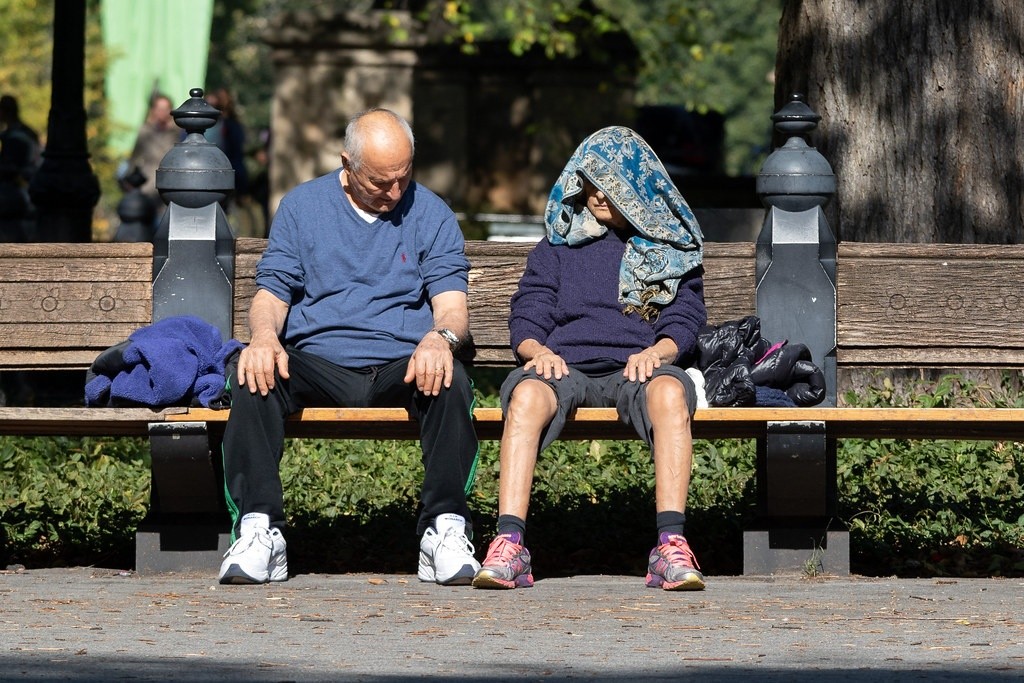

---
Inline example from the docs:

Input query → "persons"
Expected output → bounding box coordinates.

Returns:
[215,108,480,588]
[0,82,275,242]
[466,126,709,592]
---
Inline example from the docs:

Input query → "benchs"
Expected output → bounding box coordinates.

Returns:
[0,87,1023,578]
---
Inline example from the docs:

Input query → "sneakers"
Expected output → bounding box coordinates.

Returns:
[217,511,289,583]
[471,533,535,589]
[416,512,482,585]
[644,533,707,591]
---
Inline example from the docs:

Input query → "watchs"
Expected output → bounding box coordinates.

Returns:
[431,325,460,353]
